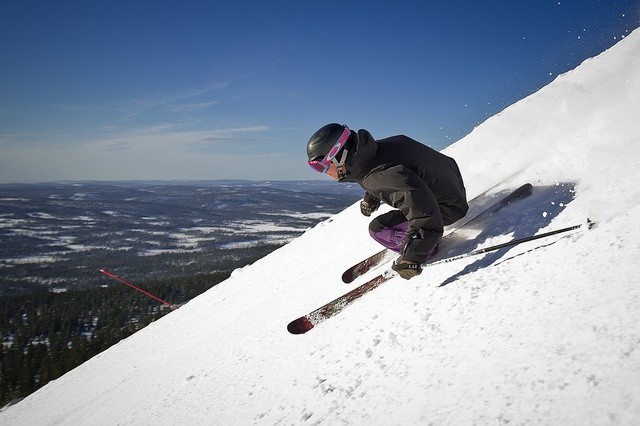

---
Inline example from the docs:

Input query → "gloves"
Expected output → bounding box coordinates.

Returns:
[360,202,376,216]
[395,258,422,280]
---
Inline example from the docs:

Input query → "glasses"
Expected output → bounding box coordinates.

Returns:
[308,125,351,174]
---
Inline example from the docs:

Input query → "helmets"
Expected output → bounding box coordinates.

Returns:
[307,123,357,176]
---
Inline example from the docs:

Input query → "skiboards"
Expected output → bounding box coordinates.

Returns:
[287,165,533,335]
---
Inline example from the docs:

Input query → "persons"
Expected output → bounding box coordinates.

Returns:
[307,122,468,280]
